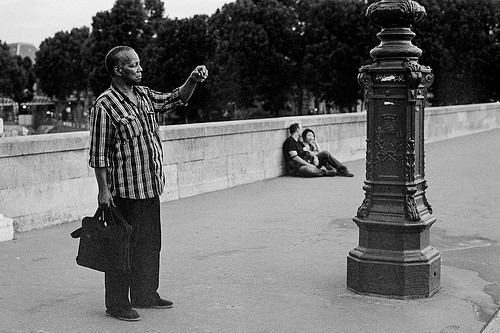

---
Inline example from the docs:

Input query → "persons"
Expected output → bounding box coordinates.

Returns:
[283,123,355,177]
[89,46,208,321]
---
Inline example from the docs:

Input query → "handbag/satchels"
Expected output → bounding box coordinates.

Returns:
[311,156,323,169]
[70,204,133,275]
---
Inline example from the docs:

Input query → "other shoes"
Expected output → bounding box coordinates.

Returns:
[105,307,141,320]
[336,166,354,177]
[326,164,333,170]
[327,170,336,176]
[131,299,174,308]
